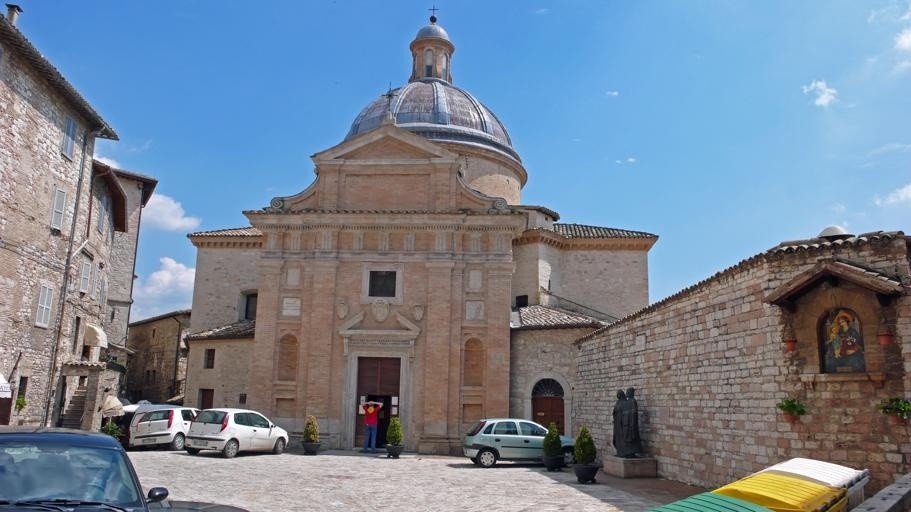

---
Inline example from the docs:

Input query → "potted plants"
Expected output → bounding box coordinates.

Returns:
[538,422,568,471]
[567,425,604,483]
[301,415,322,455]
[385,416,405,458]
[778,400,806,423]
[878,398,911,425]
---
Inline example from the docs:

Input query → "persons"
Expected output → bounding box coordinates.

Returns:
[822,308,865,373]
[359,400,384,454]
[612,389,629,458]
[618,387,642,458]
[826,321,848,362]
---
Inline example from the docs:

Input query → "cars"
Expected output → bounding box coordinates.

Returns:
[0,425,169,512]
[129,404,289,458]
[463,418,575,468]
